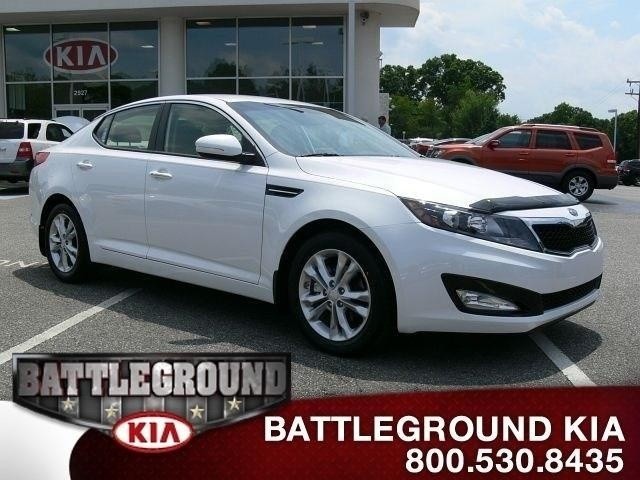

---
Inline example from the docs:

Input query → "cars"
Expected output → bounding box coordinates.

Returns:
[403,132,489,153]
[24,93,604,356]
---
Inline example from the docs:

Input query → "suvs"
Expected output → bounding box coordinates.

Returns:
[1,116,91,185]
[424,122,618,203]
[618,160,639,187]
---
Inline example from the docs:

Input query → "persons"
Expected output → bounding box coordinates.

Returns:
[376,115,393,136]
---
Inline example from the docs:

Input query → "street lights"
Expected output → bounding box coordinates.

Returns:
[609,109,617,154]
